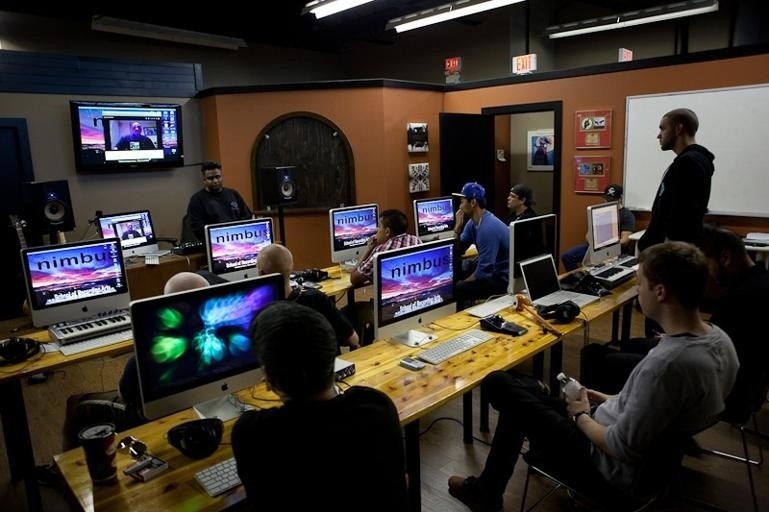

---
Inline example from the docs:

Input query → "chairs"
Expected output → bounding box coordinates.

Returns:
[676,365,763,512]
[521,420,682,511]
[62,387,146,449]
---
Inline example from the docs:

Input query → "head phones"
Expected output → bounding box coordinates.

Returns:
[559,270,613,297]
[0,336,39,367]
[535,300,580,325]
[289,267,328,285]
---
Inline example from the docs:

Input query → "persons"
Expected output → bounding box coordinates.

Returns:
[506,187,544,220]
[602,227,768,415]
[114,121,157,150]
[562,185,637,271]
[230,303,410,512]
[444,183,514,307]
[255,245,360,354]
[638,109,715,339]
[121,221,140,238]
[115,271,213,416]
[444,241,742,512]
[340,210,425,324]
[183,162,254,234]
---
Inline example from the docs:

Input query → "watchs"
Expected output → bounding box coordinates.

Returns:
[571,411,590,425]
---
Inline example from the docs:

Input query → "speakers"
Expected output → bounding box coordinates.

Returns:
[256,166,299,209]
[19,179,77,240]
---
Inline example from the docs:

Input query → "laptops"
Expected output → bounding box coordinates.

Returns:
[519,253,601,314]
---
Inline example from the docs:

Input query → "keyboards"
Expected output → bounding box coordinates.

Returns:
[137,249,171,256]
[145,255,160,265]
[582,255,643,290]
[464,294,519,318]
[416,328,495,365]
[58,328,134,356]
[193,457,243,497]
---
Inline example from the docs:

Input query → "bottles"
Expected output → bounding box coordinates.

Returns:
[557,373,599,419]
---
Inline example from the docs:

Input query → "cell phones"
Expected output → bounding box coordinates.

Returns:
[400,357,426,371]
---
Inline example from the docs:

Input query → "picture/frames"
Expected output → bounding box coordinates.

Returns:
[574,155,611,194]
[527,129,554,171]
[575,109,612,149]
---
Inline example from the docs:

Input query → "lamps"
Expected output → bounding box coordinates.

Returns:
[386,0,527,33]
[543,0,719,40]
[91,16,247,50]
[301,0,373,20]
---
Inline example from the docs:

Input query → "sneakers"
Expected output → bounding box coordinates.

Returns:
[535,378,552,401]
[448,473,505,511]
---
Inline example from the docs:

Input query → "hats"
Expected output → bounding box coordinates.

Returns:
[511,183,536,205]
[603,185,623,199]
[452,183,486,200]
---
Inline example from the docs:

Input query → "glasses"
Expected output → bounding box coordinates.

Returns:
[117,436,146,458]
[509,193,518,199]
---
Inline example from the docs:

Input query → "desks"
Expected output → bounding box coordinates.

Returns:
[0,244,481,512]
[123,243,203,301]
[628,229,769,269]
[52,256,641,512]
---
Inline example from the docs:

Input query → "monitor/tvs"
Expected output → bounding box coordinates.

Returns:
[97,209,157,258]
[413,195,457,241]
[507,214,559,295]
[69,99,184,176]
[581,201,621,267]
[129,271,286,424]
[373,236,457,348]
[20,237,131,345]
[204,217,275,283]
[329,203,379,273]
